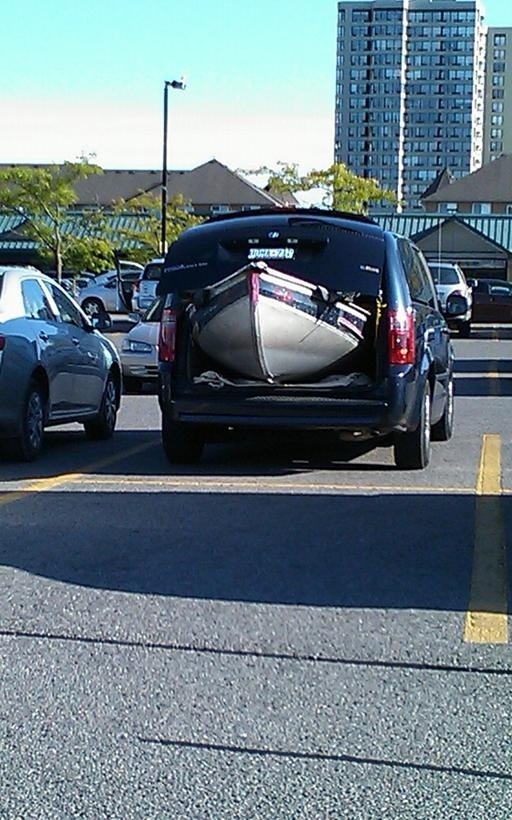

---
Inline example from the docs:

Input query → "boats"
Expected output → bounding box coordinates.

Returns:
[185,260,372,386]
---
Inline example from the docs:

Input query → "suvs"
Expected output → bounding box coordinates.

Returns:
[426,262,477,335]
[158,207,469,472]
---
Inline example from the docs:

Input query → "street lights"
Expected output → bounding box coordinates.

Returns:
[161,79,187,255]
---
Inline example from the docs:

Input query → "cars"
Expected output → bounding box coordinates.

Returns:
[44,259,166,395]
[0,264,125,460]
[468,277,512,323]
